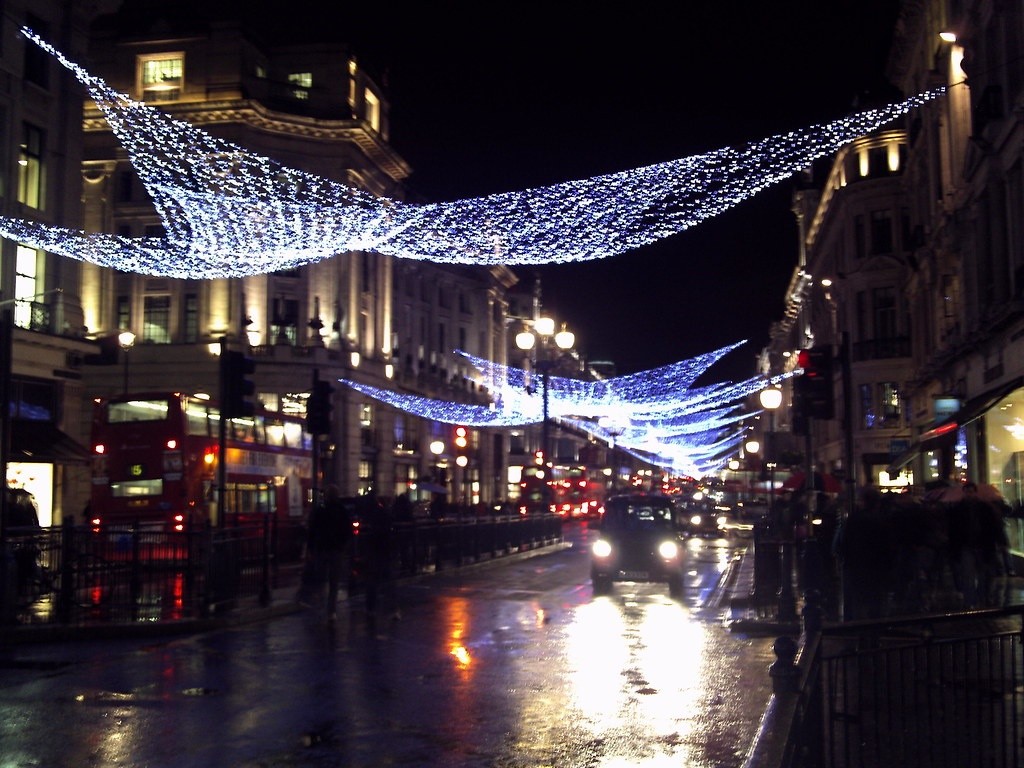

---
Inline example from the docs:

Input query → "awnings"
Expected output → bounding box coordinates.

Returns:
[420,483,448,493]
[6,417,93,466]
[885,376,1024,480]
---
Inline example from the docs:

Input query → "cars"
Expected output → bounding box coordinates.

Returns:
[670,496,719,537]
[585,492,688,584]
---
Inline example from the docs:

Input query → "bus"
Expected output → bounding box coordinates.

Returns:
[83,389,323,563]
[521,461,606,519]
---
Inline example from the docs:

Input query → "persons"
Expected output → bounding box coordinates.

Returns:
[308,484,351,617]
[775,481,1018,622]
[372,493,448,577]
[6,488,41,603]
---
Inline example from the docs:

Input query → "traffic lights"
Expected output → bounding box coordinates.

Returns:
[305,390,332,435]
[223,350,257,418]
[797,344,834,419]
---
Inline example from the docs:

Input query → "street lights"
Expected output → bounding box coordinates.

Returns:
[514,316,576,514]
[759,376,782,551]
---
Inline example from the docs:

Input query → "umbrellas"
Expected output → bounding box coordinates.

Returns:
[783,472,845,492]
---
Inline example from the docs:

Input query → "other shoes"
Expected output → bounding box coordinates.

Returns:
[386,612,401,622]
[298,599,313,609]
[328,612,338,622]
